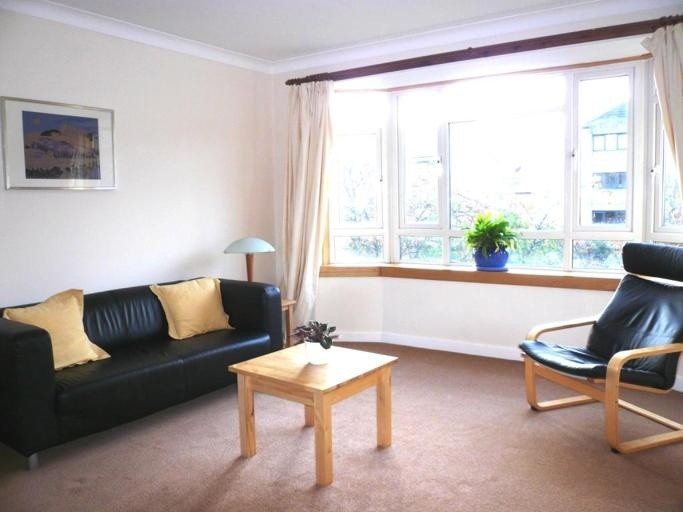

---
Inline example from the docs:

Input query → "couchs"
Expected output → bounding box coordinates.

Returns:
[0,278,282,472]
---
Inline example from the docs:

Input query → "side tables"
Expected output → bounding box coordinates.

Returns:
[281,300,297,347]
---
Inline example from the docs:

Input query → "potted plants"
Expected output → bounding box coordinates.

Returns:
[463,211,520,271]
[293,320,340,366]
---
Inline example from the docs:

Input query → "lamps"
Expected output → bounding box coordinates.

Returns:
[224,236,276,282]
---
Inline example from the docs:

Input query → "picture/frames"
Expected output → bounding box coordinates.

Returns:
[0,95,116,192]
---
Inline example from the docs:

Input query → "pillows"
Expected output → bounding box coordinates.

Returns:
[148,278,236,340]
[3,289,111,372]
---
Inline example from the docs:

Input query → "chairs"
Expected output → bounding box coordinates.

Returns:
[518,242,683,454]
